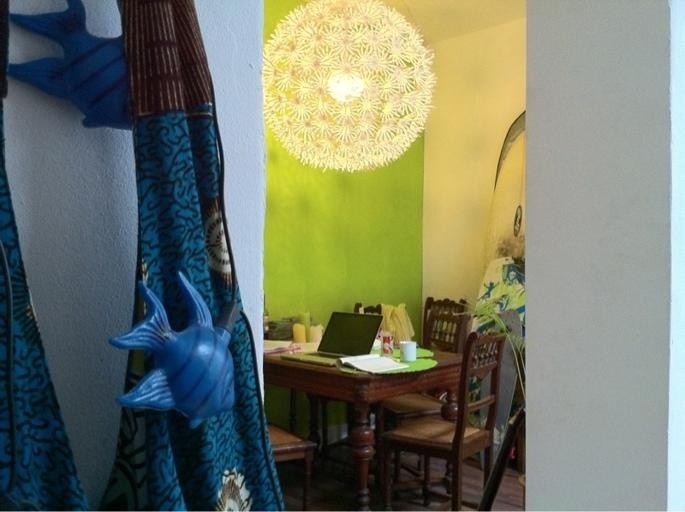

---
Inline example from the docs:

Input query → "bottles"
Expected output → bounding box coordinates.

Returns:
[262,294,269,338]
[380,331,393,358]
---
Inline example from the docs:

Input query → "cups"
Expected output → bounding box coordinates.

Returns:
[399,340,416,362]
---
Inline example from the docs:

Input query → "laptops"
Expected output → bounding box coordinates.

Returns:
[280,311,384,367]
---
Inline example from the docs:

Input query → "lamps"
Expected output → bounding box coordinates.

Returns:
[261,2,436,175]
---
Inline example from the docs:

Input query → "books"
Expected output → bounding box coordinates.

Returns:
[336,353,410,376]
